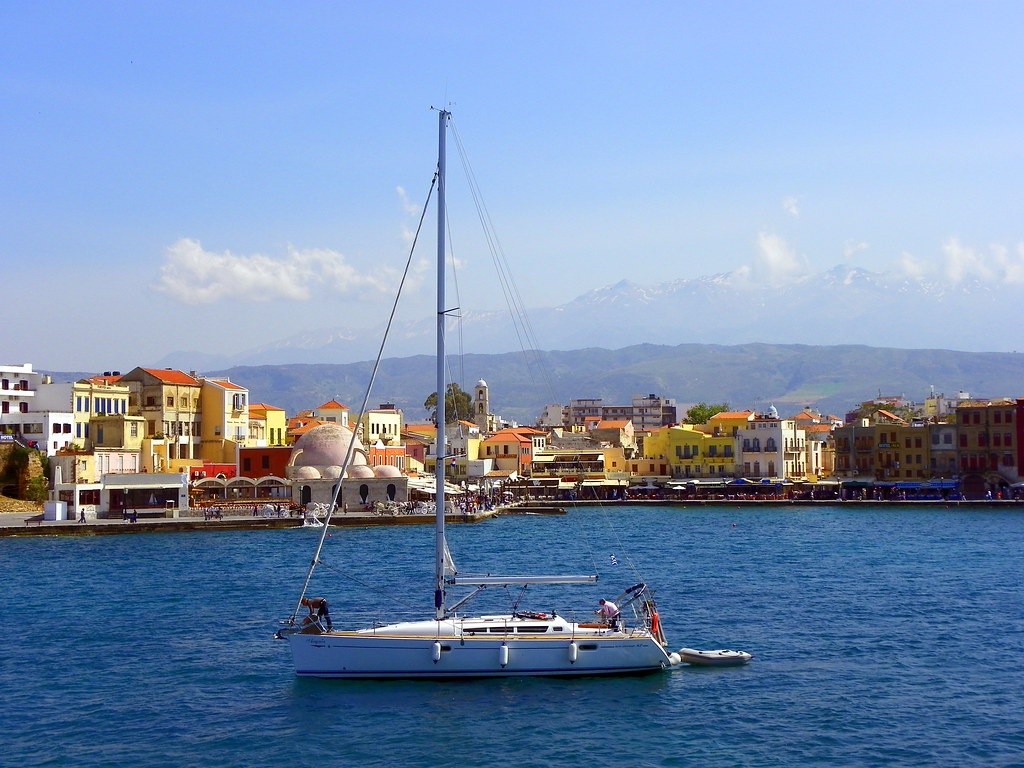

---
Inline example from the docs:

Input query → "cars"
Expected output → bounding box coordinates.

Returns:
[0,431,40,454]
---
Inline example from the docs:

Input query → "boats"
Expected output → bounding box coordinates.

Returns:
[678,647,756,667]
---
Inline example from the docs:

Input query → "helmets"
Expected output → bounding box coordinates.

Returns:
[300,597,308,603]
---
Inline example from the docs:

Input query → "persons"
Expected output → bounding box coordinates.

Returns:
[204,504,223,520]
[78,508,86,523]
[810,490,814,500]
[986,488,1024,500]
[133,510,137,522]
[859,486,905,501]
[736,492,739,499]
[344,502,348,515]
[277,504,280,517]
[603,488,628,501]
[458,492,509,515]
[253,503,258,516]
[301,597,334,633]
[595,599,621,628]
[366,499,418,515]
[962,492,966,501]
[290,504,306,517]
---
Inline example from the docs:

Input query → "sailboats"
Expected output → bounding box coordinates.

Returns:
[272,100,682,682]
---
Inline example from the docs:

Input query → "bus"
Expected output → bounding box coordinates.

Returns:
[529,459,608,483]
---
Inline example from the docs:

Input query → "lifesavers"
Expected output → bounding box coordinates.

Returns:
[652,613,658,632]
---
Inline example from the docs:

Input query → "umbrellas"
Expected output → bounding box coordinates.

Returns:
[628,485,659,494]
[673,485,686,496]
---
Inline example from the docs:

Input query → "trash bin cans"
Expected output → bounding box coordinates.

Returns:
[839,489,846,501]
[166,500,175,518]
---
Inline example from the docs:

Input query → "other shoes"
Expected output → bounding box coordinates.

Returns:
[327,626,333,633]
